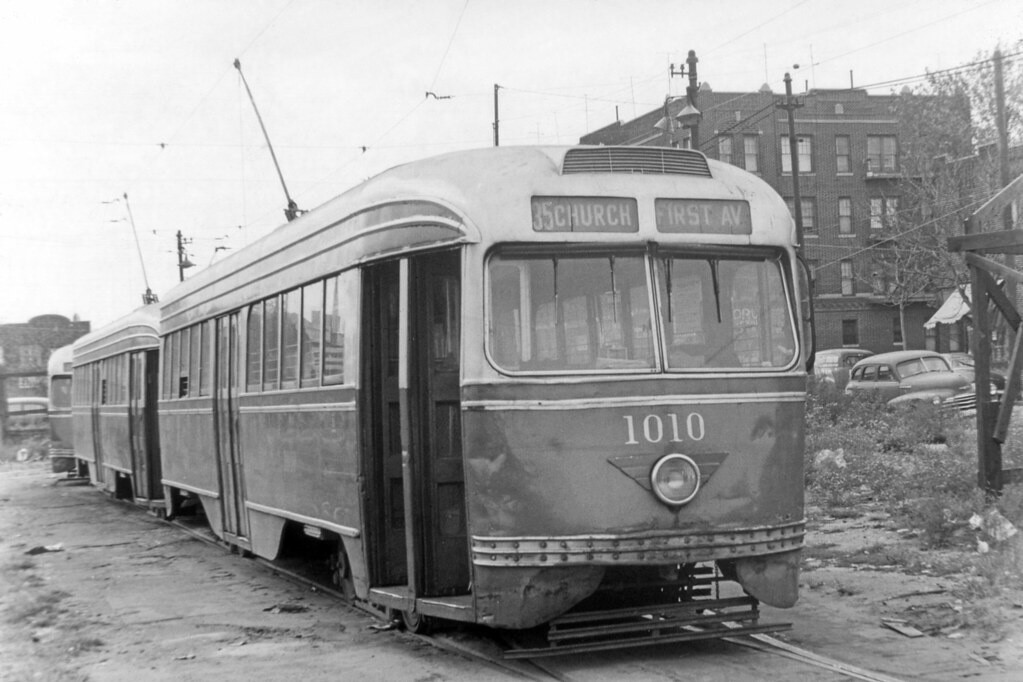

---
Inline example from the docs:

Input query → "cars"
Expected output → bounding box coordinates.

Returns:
[808,348,1006,426]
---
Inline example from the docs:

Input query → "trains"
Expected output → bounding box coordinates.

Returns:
[49,141,811,662]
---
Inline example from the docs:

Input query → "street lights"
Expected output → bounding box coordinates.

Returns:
[655,49,704,150]
